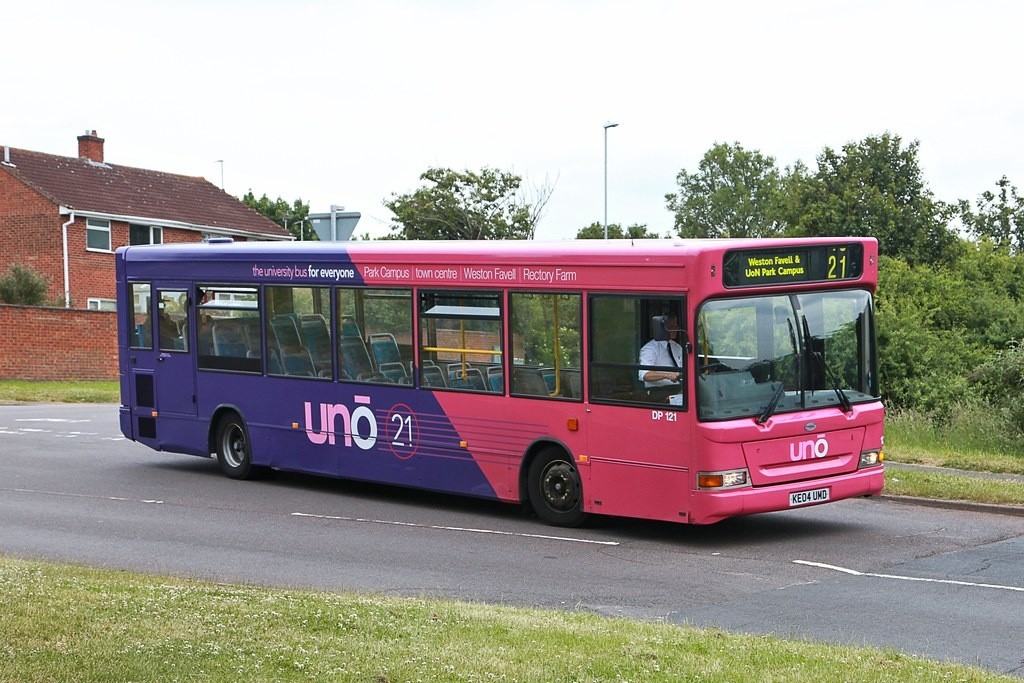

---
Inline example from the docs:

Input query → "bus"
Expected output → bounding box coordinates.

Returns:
[115,235,892,530]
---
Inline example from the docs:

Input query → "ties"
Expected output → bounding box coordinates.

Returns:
[667,342,678,367]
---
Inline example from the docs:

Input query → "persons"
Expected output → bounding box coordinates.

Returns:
[142,297,222,354]
[637,307,682,404]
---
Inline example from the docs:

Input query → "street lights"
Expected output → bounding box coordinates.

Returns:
[603,120,621,242]
[218,159,225,191]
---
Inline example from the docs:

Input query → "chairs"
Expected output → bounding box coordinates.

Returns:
[137,314,565,396]
